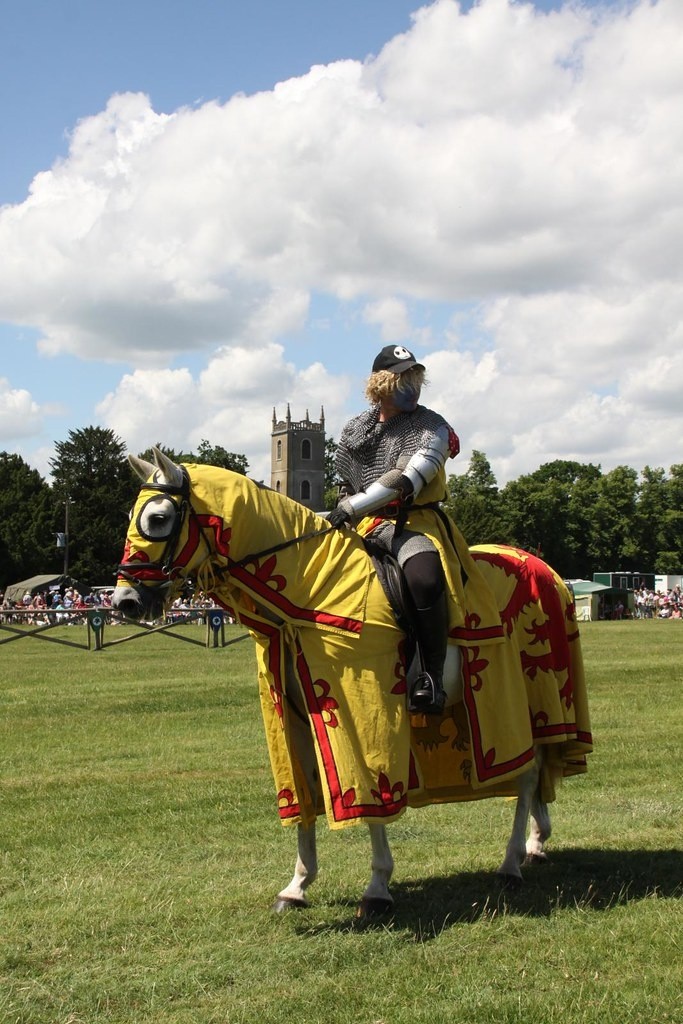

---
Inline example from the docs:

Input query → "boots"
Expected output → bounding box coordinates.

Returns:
[410,591,448,716]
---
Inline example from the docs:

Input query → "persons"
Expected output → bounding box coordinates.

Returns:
[604,584,683,620]
[325,344,459,715]
[0,587,236,625]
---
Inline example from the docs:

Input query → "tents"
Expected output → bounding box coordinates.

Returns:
[2,574,92,606]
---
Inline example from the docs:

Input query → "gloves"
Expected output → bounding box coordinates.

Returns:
[324,506,351,529]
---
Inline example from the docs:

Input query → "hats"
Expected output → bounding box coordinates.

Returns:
[371,344,426,374]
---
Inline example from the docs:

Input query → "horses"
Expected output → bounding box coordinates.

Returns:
[109,444,597,921]
